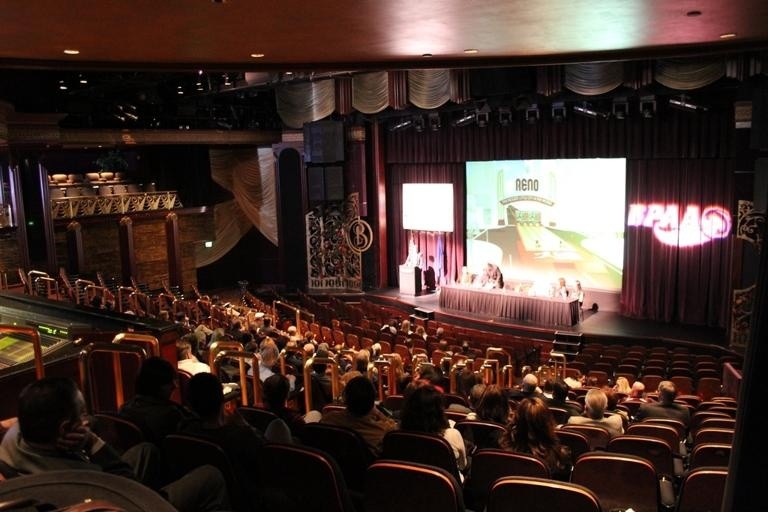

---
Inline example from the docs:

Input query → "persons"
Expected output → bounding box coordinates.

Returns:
[403,245,422,271]
[0,272,696,512]
[481,263,505,290]
